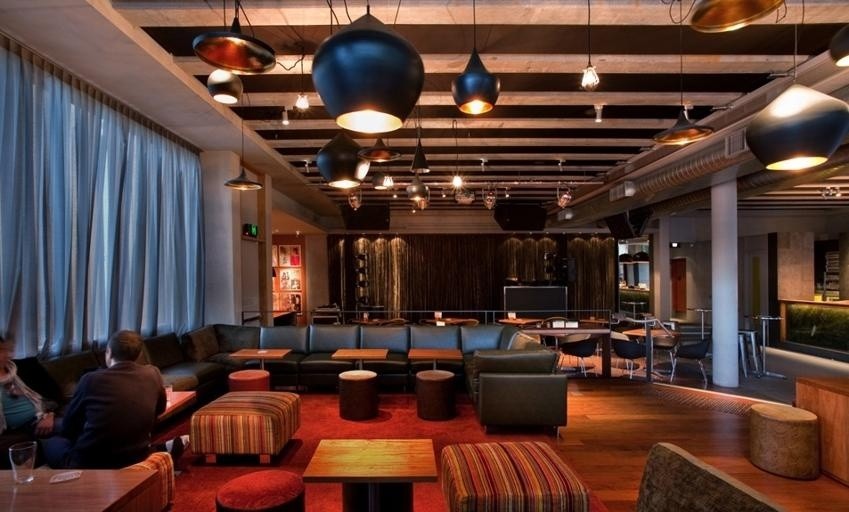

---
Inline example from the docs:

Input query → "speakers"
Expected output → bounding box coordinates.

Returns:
[493,204,548,232]
[341,204,390,231]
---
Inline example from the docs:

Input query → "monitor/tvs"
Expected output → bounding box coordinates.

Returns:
[505,285,568,321]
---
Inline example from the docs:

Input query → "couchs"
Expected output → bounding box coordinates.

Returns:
[247,323,505,391]
[464,326,569,440]
[46,326,260,422]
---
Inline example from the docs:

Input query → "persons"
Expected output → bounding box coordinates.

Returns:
[0,333,45,470]
[33,330,168,468]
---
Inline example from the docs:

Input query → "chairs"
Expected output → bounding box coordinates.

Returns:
[558,337,598,377]
[668,336,709,390]
[541,317,569,329]
[612,338,647,379]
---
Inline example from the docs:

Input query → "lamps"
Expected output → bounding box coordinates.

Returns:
[192,1,276,74]
[312,0,424,136]
[376,163,397,192]
[453,188,476,205]
[503,190,509,197]
[554,182,573,209]
[651,0,715,146]
[451,2,501,116]
[746,25,848,171]
[280,107,292,125]
[481,183,497,210]
[578,0,602,90]
[411,100,432,174]
[619,245,633,265]
[303,160,311,171]
[204,68,242,104]
[557,159,565,171]
[292,42,310,112]
[225,107,263,192]
[407,178,426,211]
[357,135,401,163]
[314,129,371,190]
[830,23,849,68]
[687,1,783,33]
[633,246,650,263]
[449,120,463,188]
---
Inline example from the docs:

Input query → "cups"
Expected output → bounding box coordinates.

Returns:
[9,441,39,483]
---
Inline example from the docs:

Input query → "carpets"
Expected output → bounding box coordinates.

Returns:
[158,391,610,511]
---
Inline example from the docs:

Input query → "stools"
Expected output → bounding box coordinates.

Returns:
[101,451,180,509]
[749,402,821,478]
[443,440,589,512]
[216,471,304,512]
[227,370,270,394]
[339,370,377,419]
[190,391,300,464]
[417,370,455,419]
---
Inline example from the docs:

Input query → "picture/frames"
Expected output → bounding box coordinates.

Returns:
[271,267,280,292]
[272,244,278,267]
[279,292,303,313]
[277,267,302,292]
[273,292,280,312]
[278,243,303,268]
